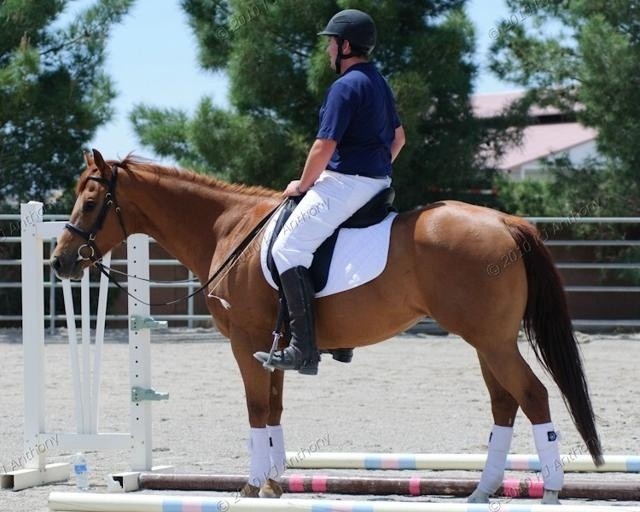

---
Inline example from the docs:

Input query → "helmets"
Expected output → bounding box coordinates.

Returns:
[316,9,379,50]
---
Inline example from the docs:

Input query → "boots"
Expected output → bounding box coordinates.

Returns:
[319,349,354,363]
[252,265,319,376]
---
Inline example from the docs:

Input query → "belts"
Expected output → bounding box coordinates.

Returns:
[351,173,391,180]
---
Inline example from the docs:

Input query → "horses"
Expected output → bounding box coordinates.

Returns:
[49,149,607,505]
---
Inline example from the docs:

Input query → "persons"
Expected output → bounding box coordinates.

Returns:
[252,9,406,375]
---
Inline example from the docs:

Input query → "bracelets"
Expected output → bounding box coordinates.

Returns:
[296,186,304,195]
